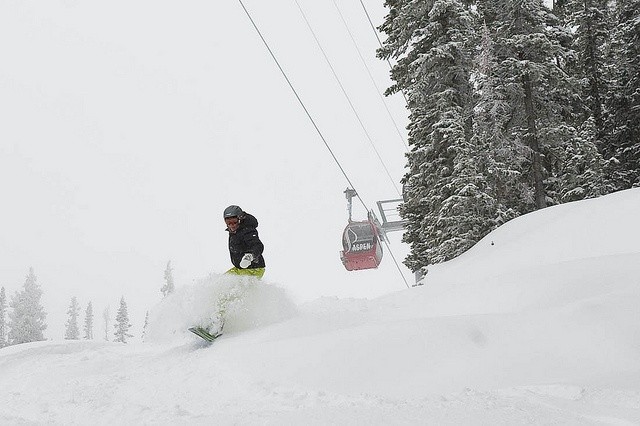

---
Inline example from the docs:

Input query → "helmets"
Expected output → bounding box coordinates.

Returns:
[224,205,246,220]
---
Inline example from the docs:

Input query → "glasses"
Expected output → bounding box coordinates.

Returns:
[225,217,238,225]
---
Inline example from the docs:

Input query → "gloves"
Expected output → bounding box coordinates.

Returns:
[240,253,253,269]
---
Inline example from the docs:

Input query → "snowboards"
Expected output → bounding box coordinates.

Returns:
[188,325,222,342]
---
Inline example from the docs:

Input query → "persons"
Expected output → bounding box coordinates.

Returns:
[206,206,266,336]
